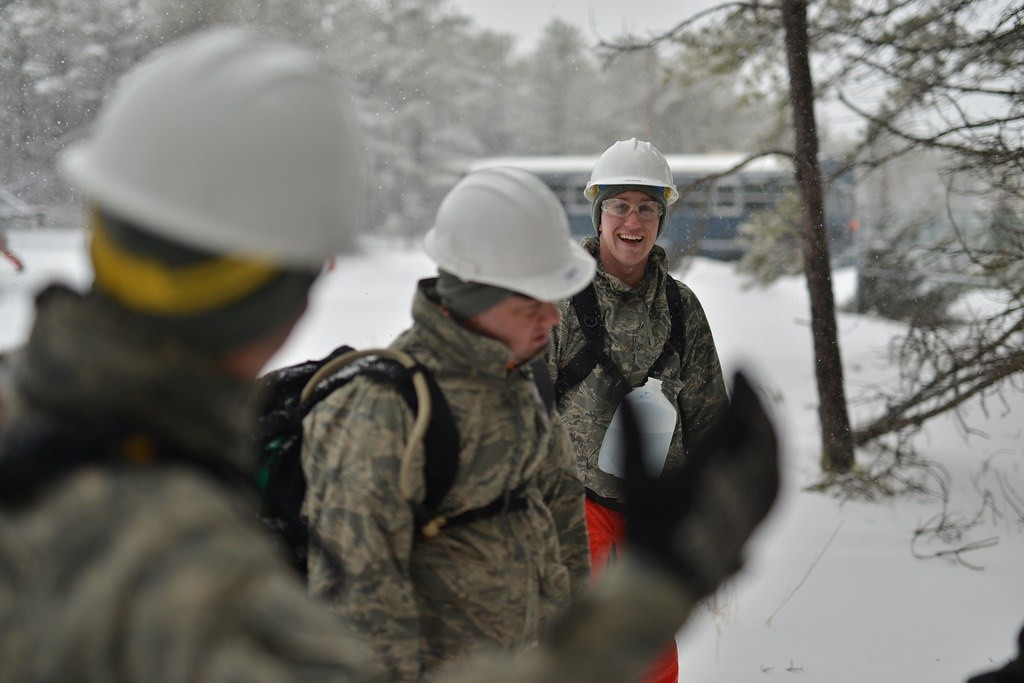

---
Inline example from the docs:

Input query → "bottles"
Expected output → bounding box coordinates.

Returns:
[597,377,678,483]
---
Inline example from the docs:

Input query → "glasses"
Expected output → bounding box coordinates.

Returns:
[601,198,664,220]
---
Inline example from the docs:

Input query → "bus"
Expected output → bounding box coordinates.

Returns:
[462,145,859,262]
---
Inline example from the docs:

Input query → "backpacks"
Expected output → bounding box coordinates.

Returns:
[243,346,556,582]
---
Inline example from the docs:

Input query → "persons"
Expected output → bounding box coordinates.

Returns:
[298,165,593,683]
[1,26,781,683]
[546,139,729,682]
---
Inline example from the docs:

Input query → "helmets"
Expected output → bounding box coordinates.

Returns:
[423,168,597,304]
[584,138,679,207]
[56,26,372,273]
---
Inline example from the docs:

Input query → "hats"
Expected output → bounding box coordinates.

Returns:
[591,185,670,239]
[435,268,511,324]
